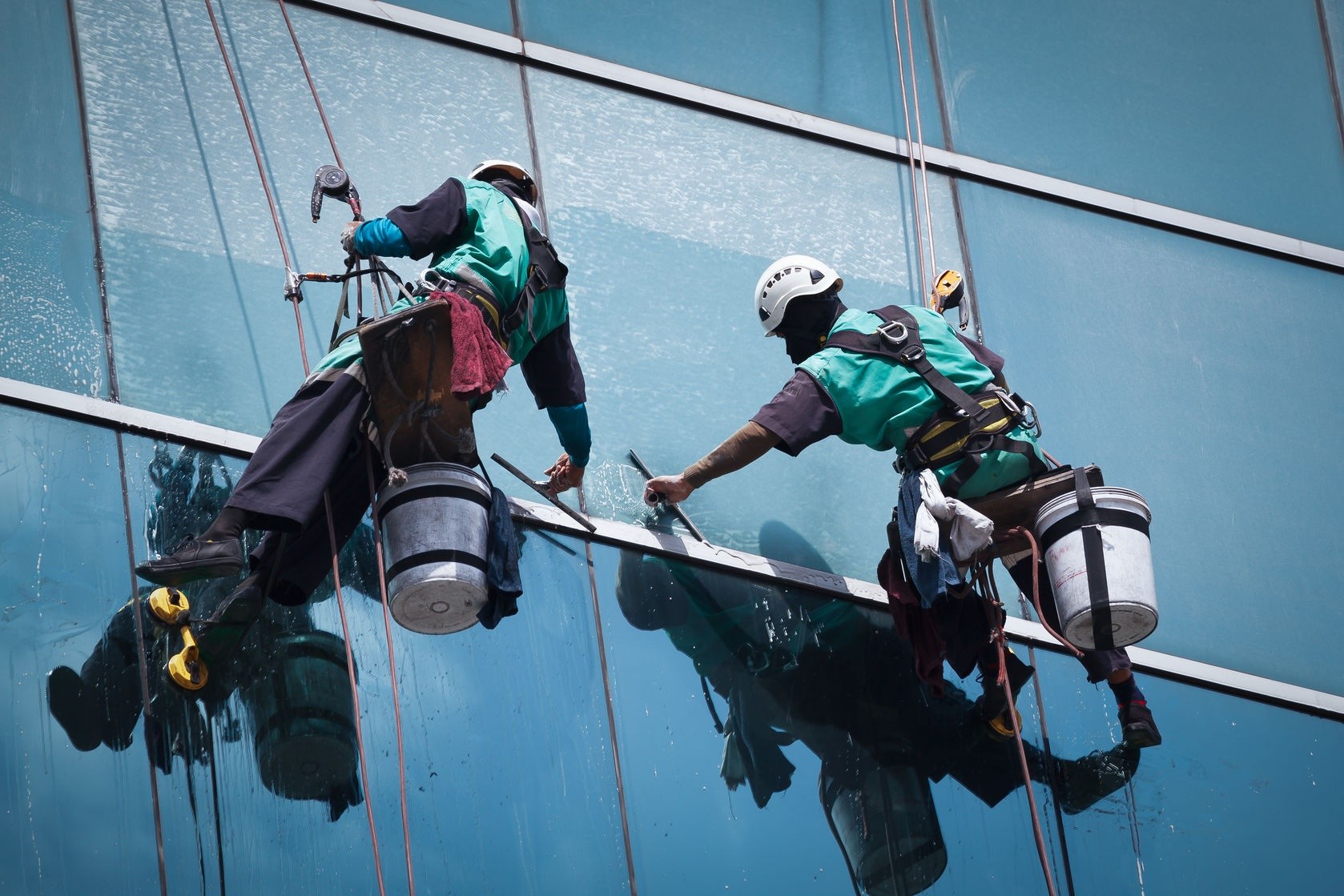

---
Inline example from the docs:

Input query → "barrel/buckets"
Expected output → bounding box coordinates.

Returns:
[240,632,358,799]
[373,462,492,636]
[817,741,948,896]
[1035,487,1157,649]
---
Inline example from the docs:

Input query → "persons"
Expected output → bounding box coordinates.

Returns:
[617,510,1141,816]
[135,159,592,672]
[644,253,1162,748]
[45,487,389,775]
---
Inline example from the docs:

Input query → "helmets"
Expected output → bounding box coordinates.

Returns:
[467,159,538,209]
[755,254,845,338]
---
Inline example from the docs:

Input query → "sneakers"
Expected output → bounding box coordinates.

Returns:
[46,664,133,750]
[1063,744,1141,816]
[978,655,1033,715]
[134,539,242,586]
[1116,704,1163,747]
[207,588,259,650]
